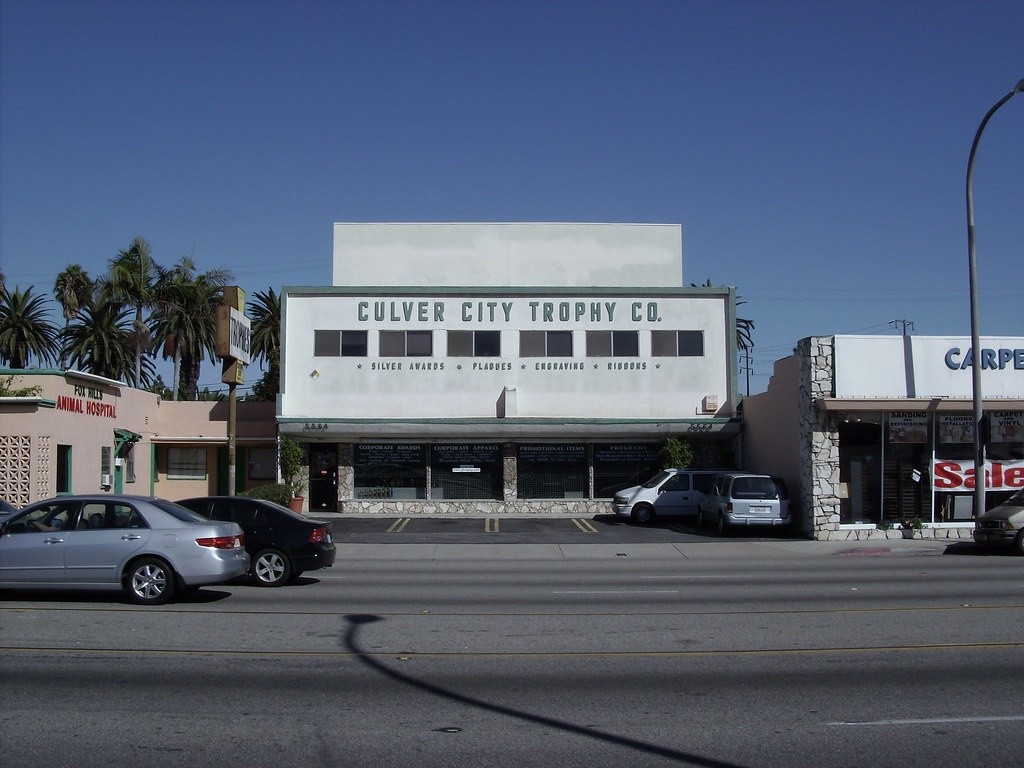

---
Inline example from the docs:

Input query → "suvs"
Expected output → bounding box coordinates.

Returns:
[698,473,793,536]
[595,464,663,499]
[610,468,753,526]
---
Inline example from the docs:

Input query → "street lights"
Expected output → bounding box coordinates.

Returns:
[969,77,1024,530]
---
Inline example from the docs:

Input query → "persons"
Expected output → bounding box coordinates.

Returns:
[26,505,76,531]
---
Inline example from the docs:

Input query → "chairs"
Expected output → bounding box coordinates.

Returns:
[89,513,104,529]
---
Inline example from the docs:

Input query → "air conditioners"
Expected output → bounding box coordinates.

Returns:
[102,474,113,486]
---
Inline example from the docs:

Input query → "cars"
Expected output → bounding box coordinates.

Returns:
[0,494,250,606]
[116,496,336,588]
[973,486,1024,555]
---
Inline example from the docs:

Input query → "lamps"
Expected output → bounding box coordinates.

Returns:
[140,431,160,437]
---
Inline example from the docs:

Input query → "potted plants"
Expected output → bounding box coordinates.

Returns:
[274,434,308,513]
